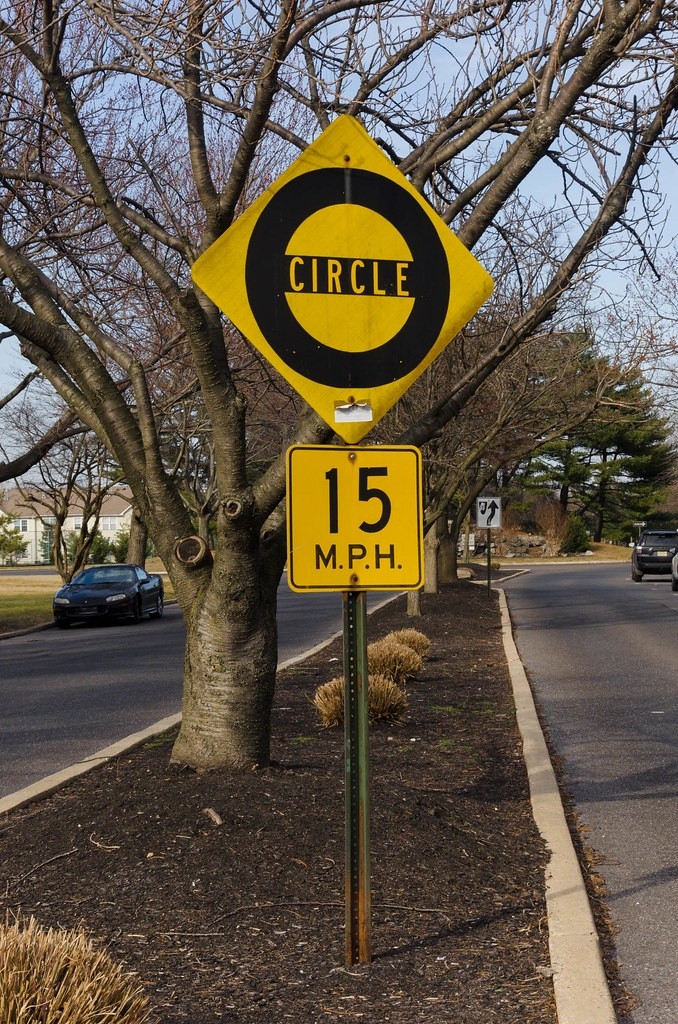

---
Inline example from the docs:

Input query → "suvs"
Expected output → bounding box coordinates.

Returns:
[631,528,678,582]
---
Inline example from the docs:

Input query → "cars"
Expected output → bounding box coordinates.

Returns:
[672,553,678,591]
[53,564,164,629]
